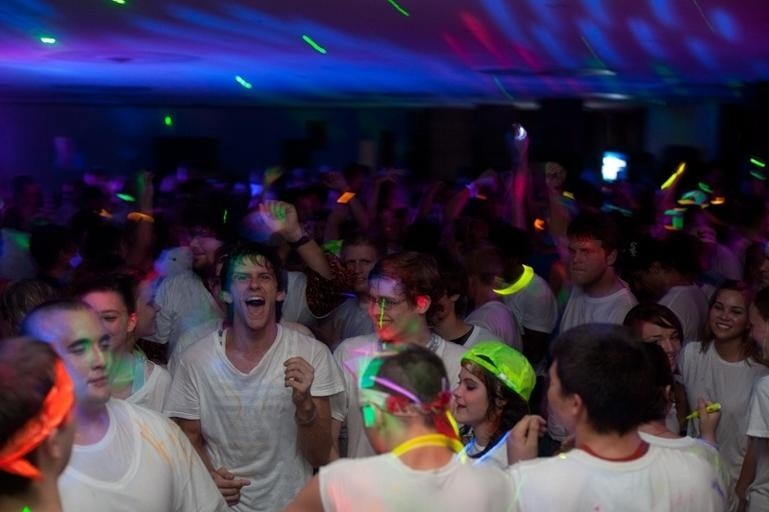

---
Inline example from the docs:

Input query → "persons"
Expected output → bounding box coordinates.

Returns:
[1,164,769,512]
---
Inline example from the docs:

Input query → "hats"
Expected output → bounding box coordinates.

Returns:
[461,341,537,414]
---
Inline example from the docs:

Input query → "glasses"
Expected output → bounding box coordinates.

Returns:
[365,295,405,311]
